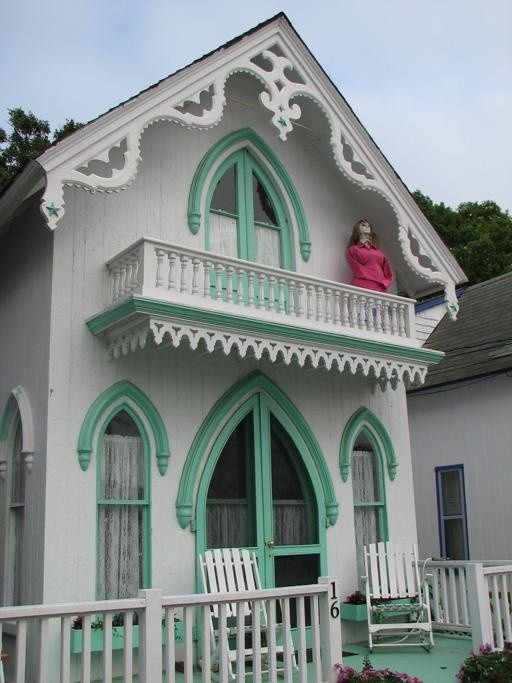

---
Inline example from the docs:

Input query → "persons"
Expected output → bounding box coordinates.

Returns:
[347,219,392,291]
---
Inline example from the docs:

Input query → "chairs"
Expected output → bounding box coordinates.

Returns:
[362,538,435,654]
[199,547,300,683]
